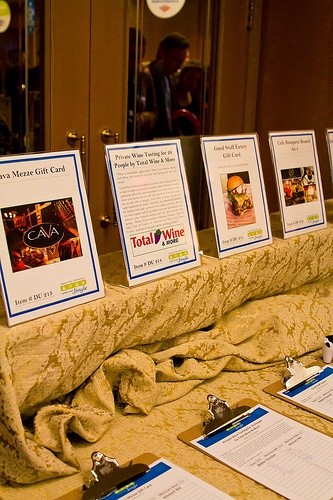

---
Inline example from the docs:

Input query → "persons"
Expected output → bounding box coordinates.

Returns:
[3,50,27,143]
[127,26,203,142]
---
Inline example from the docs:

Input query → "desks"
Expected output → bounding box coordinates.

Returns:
[0,198,333,489]
